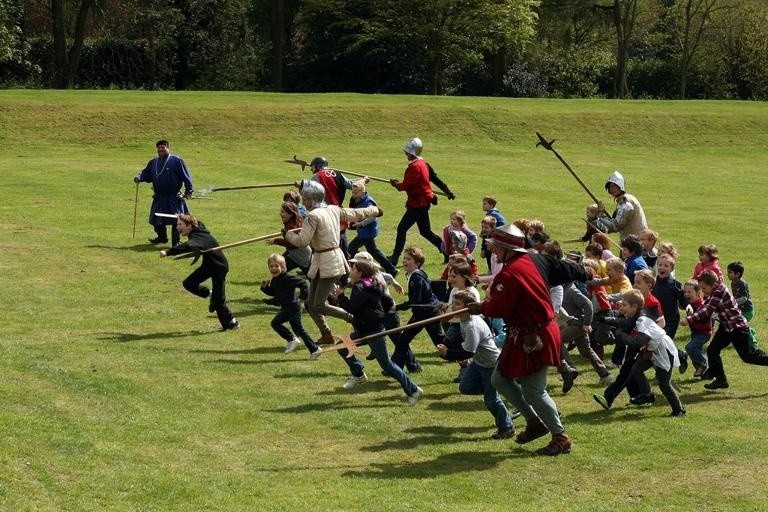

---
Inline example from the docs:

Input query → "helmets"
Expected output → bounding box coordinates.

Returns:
[310,158,327,169]
[605,170,626,195]
[298,180,329,209]
[484,223,531,254]
[405,137,424,158]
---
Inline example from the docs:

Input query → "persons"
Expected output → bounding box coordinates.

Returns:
[256,156,768,457]
[157,213,241,333]
[134,138,194,248]
[384,135,456,264]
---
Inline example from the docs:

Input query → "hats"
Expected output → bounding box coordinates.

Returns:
[353,179,366,194]
[157,140,169,147]
[348,252,373,264]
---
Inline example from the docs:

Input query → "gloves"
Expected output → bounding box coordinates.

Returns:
[448,192,456,200]
[390,178,400,187]
[378,208,384,218]
[585,265,596,281]
[467,302,482,315]
[281,228,288,237]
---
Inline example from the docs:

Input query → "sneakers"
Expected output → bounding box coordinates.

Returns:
[384,255,399,283]
[566,331,615,360]
[453,368,467,383]
[593,393,609,409]
[262,297,280,308]
[489,409,521,439]
[627,391,656,408]
[408,387,423,407]
[284,337,300,354]
[223,321,240,332]
[209,302,215,313]
[694,363,730,389]
[315,332,333,345]
[679,351,688,373]
[344,313,356,330]
[670,410,687,417]
[309,348,321,360]
[148,237,168,244]
[366,352,423,375]
[343,374,367,388]
[596,360,622,388]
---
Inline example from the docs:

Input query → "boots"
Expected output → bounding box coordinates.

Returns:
[515,415,549,444]
[536,430,571,456]
[557,361,578,393]
